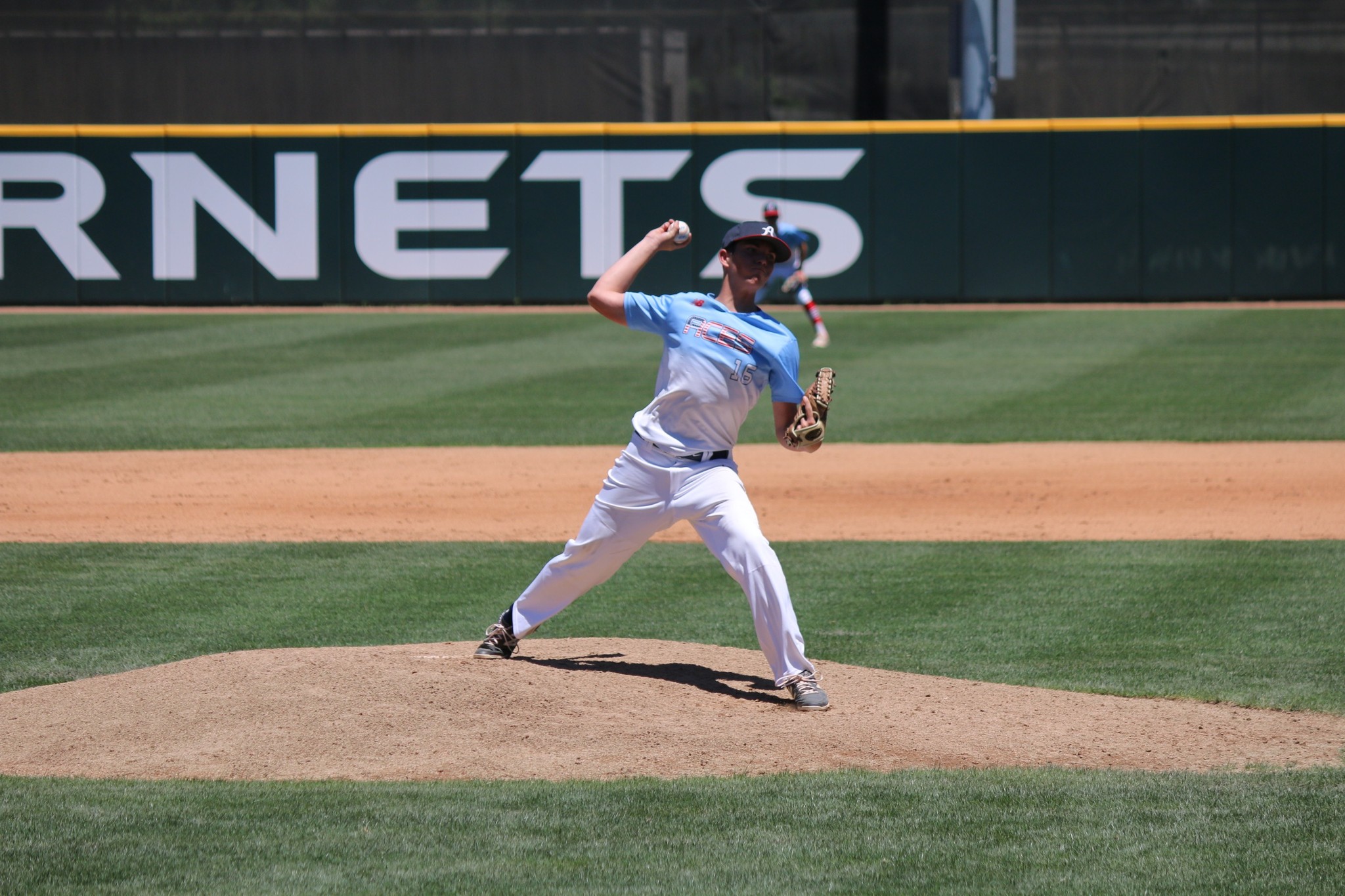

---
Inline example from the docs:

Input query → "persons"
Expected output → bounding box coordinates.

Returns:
[753,200,832,350]
[472,218,836,712]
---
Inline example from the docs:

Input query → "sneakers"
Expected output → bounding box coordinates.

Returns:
[774,668,831,712]
[473,609,520,661]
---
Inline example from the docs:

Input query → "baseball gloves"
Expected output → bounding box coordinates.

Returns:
[780,275,801,292]
[784,367,835,448]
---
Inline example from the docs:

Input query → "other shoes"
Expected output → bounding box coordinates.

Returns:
[810,331,833,350]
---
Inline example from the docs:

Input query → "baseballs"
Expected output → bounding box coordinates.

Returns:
[668,221,689,245]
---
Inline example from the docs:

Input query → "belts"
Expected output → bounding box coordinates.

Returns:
[634,429,729,463]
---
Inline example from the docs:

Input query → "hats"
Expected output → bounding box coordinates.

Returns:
[721,221,792,263]
[763,202,780,217]
